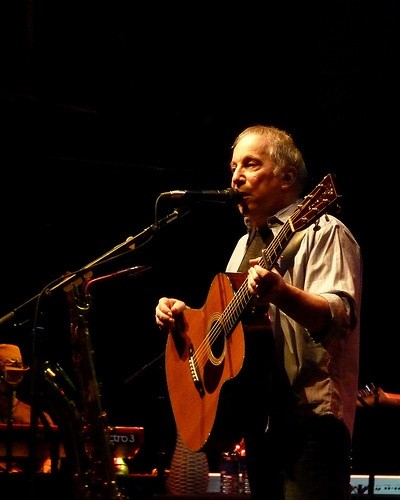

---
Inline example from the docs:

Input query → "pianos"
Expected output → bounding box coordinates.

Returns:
[0,424,144,461]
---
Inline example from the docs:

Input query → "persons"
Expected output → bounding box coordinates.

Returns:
[156,125,360,500]
[0,343,66,474]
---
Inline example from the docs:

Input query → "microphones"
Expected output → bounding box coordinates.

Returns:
[162,187,243,206]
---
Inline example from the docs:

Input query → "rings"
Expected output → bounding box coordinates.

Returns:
[256,285,259,287]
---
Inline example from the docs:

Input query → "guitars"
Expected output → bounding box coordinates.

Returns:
[163,172,337,454]
